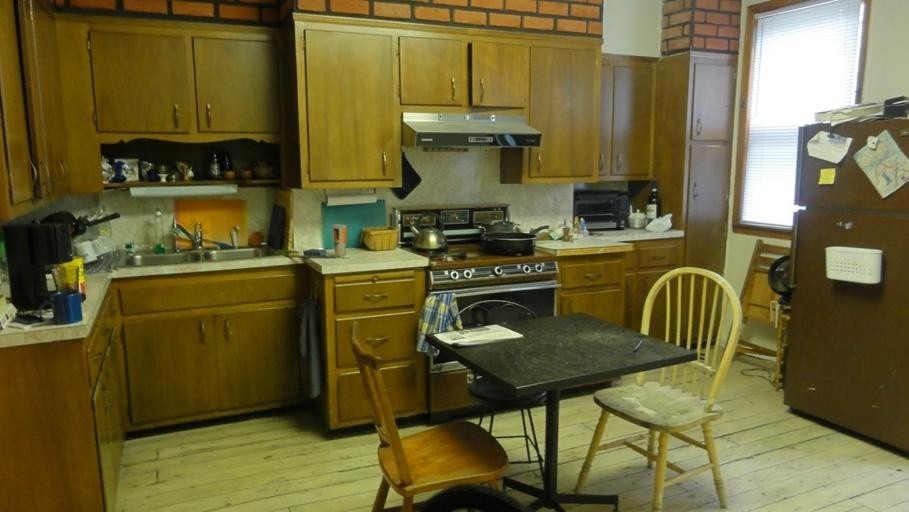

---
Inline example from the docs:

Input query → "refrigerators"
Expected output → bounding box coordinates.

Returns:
[785,116,909,456]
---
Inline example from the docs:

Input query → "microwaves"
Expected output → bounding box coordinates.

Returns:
[574,192,627,231]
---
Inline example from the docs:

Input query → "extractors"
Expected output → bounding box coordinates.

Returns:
[401,111,542,149]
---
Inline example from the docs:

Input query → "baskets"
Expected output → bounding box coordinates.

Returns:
[360,226,401,252]
[824,245,883,285]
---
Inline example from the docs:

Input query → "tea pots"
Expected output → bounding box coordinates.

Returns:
[411,210,448,253]
[72,239,104,266]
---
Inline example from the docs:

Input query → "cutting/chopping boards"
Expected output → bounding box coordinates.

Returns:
[267,188,295,252]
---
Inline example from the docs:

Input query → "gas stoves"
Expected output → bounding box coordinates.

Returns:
[405,238,555,268]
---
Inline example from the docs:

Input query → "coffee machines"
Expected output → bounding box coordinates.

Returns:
[5,219,74,308]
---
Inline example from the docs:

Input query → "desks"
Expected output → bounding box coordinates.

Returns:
[425,311,700,512]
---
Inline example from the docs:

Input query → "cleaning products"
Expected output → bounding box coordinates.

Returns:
[154,208,165,253]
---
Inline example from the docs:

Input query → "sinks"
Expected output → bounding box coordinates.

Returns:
[117,251,200,267]
[203,248,265,260]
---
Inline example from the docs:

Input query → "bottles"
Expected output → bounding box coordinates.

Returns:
[573,217,589,236]
[208,153,222,180]
[645,187,660,223]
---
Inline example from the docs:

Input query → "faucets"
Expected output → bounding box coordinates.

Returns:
[171,217,204,249]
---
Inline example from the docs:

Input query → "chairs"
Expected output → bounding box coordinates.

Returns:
[734,239,793,390]
[347,317,510,511]
[574,265,741,511]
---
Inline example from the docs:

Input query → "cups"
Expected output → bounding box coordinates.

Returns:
[51,289,83,324]
[333,224,346,256]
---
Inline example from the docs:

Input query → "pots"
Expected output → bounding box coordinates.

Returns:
[475,218,551,257]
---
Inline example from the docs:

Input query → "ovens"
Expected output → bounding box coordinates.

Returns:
[427,279,564,373]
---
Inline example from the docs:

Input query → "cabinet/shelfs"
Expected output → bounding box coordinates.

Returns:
[657,50,740,351]
[601,52,654,187]
[291,12,403,191]
[402,18,528,115]
[559,229,684,327]
[117,268,308,435]
[521,33,606,187]
[60,8,284,195]
[326,248,430,429]
[1,1,58,222]
[0,272,129,511]
